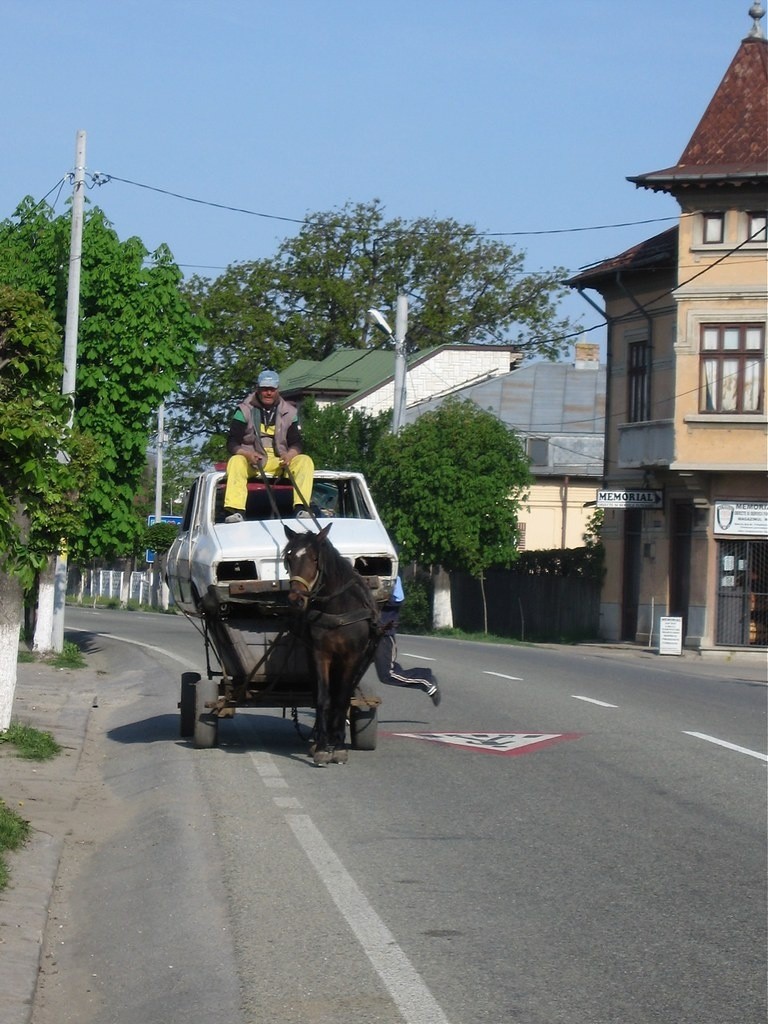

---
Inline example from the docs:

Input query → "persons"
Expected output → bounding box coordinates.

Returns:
[223,370,316,524]
[357,577,441,706]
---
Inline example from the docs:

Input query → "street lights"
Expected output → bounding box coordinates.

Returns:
[366,294,409,436]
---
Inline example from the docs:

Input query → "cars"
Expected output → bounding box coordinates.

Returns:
[164,458,400,614]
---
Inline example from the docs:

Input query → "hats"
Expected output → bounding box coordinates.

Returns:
[258,370,279,388]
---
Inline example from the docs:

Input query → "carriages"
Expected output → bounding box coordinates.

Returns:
[177,519,400,768]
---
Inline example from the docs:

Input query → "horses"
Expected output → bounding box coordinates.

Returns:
[280,523,382,767]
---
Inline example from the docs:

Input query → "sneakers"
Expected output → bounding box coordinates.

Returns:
[296,510,316,518]
[225,512,244,521]
